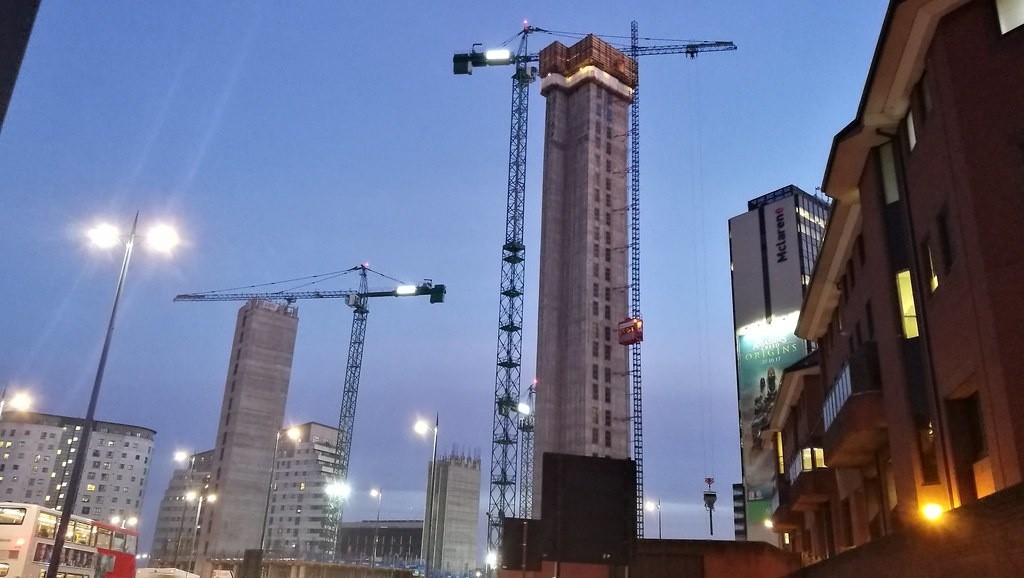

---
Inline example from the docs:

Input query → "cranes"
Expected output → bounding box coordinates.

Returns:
[173,262,449,559]
[452,25,740,566]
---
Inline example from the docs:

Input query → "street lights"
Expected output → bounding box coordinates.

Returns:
[187,493,215,571]
[173,453,194,567]
[259,428,300,549]
[647,502,662,538]
[415,421,437,578]
[0,396,29,419]
[371,490,381,565]
[40,226,180,578]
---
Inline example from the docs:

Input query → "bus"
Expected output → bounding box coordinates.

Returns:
[1,502,140,578]
